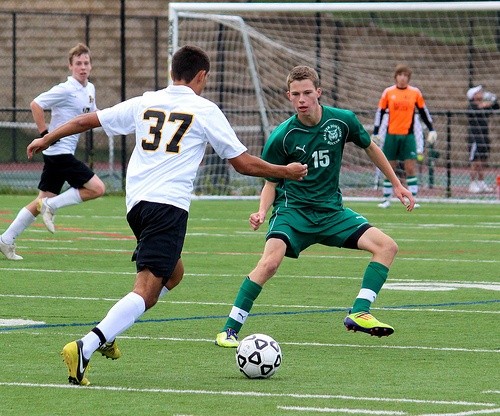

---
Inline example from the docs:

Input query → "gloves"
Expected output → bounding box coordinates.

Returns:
[370,134,381,147]
[426,131,438,145]
[39,128,61,147]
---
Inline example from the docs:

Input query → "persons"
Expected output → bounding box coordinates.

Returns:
[27,45,308,385]
[0,42,106,260]
[373,63,437,209]
[464,85,500,193]
[215,66,415,348]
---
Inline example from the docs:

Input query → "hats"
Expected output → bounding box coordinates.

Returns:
[466,85,482,100]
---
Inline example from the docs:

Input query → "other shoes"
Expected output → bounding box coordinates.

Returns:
[376,200,392,208]
[468,182,494,194]
[413,203,420,209]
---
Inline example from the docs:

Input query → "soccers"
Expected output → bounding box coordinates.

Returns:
[235,334,282,379]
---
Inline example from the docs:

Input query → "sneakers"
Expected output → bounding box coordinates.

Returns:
[0,234,24,261]
[343,310,395,338]
[60,338,91,386]
[95,338,121,361]
[214,328,239,349]
[35,197,55,235]
